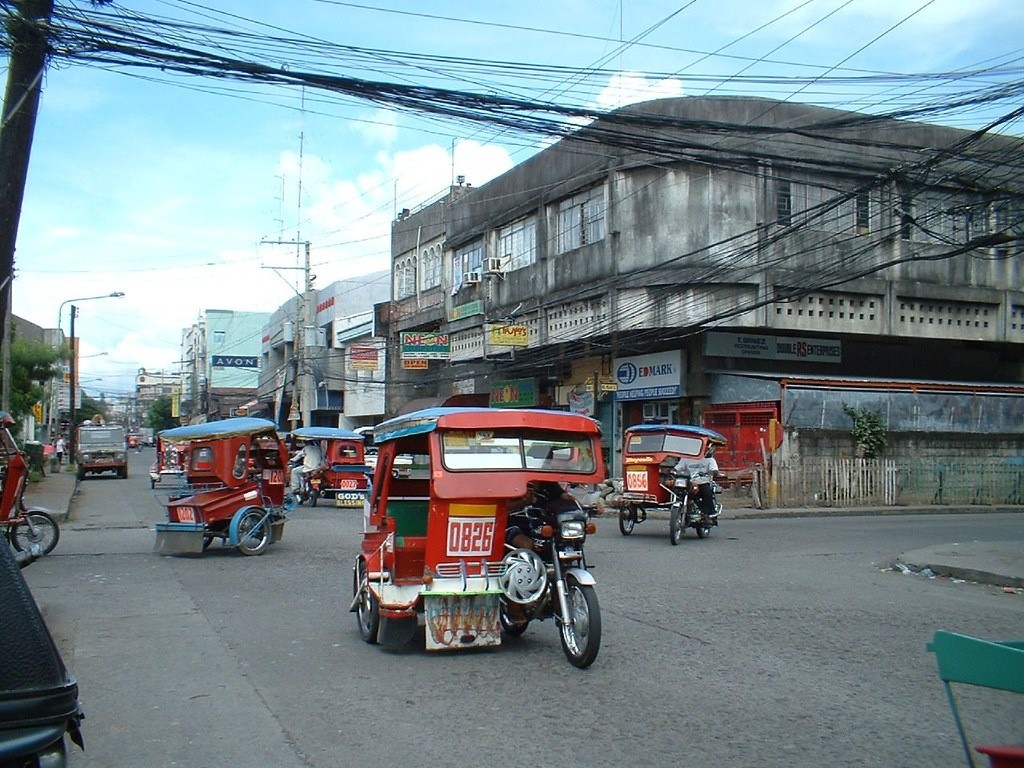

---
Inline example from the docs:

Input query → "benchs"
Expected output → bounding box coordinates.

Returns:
[239,449,282,469]
[369,497,430,553]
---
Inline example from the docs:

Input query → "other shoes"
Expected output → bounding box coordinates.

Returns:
[291,491,301,494]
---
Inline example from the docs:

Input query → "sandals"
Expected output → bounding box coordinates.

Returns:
[504,604,527,624]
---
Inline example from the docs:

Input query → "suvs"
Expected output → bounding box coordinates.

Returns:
[77,425,129,481]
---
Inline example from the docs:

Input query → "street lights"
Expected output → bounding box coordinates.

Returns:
[56,291,125,464]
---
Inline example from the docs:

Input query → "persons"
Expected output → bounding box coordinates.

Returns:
[290,441,329,498]
[502,444,601,624]
[662,440,719,528]
[50,432,69,467]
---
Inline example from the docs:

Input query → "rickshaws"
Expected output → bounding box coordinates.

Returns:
[156,414,291,558]
[286,427,372,509]
[0,411,62,558]
[350,407,604,671]
[604,424,729,546]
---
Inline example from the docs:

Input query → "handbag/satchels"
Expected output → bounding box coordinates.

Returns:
[321,450,331,471]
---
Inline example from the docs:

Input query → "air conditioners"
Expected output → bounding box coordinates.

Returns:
[481,257,508,273]
[463,272,482,284]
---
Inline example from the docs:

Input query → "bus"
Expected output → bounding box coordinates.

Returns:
[127,426,156,448]
